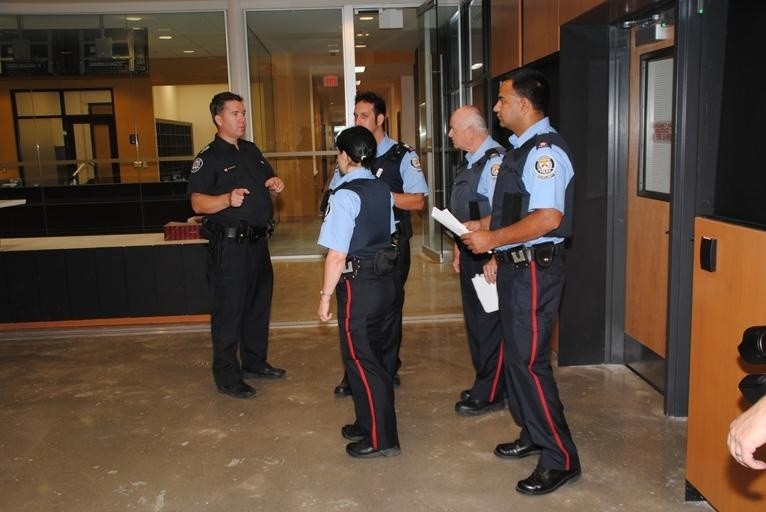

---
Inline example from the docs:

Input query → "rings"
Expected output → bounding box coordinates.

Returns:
[735,452,741,457]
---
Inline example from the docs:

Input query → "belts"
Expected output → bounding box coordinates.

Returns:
[495,242,566,264]
[221,226,266,239]
[341,259,374,274]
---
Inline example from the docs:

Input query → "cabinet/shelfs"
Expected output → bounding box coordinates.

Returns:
[155,118,196,182]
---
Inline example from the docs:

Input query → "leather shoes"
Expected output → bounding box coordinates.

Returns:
[217,381,257,399]
[516,466,581,495]
[241,360,286,378]
[335,371,352,395]
[393,373,400,386]
[346,436,401,459]
[455,398,505,416]
[461,389,509,408]
[494,439,543,459]
[342,424,370,441]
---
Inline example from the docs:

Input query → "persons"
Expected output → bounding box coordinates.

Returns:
[334,90,430,398]
[189,92,287,399]
[317,126,403,460]
[448,106,508,415]
[726,393,766,470]
[458,69,584,499]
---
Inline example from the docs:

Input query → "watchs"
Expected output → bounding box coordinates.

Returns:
[319,288,332,295]
